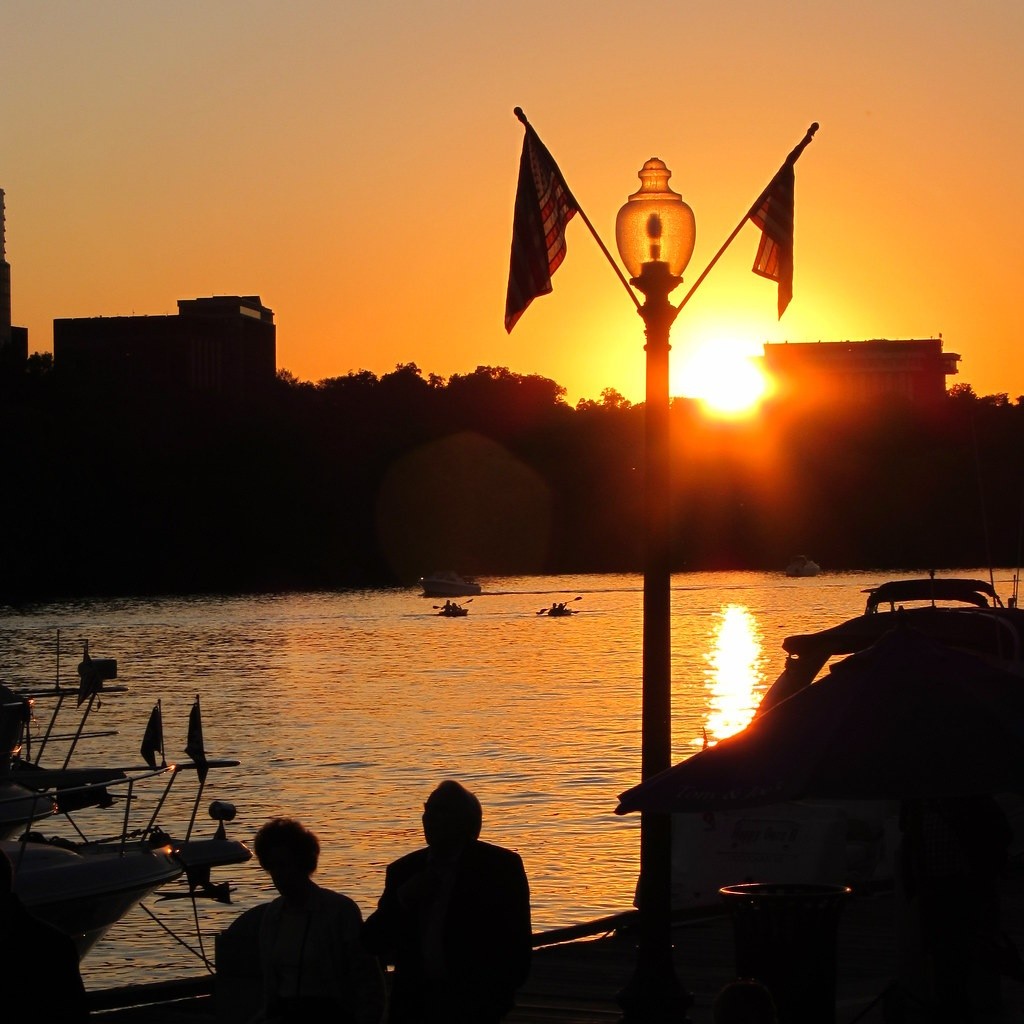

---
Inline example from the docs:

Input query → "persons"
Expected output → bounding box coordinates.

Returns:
[366,780,531,1024]
[448,603,462,612]
[441,600,452,612]
[897,746,1024,1010]
[549,602,567,613]
[712,981,782,1024]
[210,819,386,1024]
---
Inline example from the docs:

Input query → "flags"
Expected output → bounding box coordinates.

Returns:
[140,706,163,772]
[77,656,102,708]
[184,701,209,785]
[750,157,795,320]
[504,127,579,335]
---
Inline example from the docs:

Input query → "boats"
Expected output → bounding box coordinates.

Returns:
[419,570,482,597]
[438,609,469,616]
[766,574,1004,707]
[549,609,574,615]
[1,636,251,1023]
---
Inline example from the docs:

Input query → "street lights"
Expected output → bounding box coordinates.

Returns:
[613,155,701,989]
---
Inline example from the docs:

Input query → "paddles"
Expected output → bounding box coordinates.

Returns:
[432,605,443,609]
[458,598,474,606]
[540,596,582,612]
